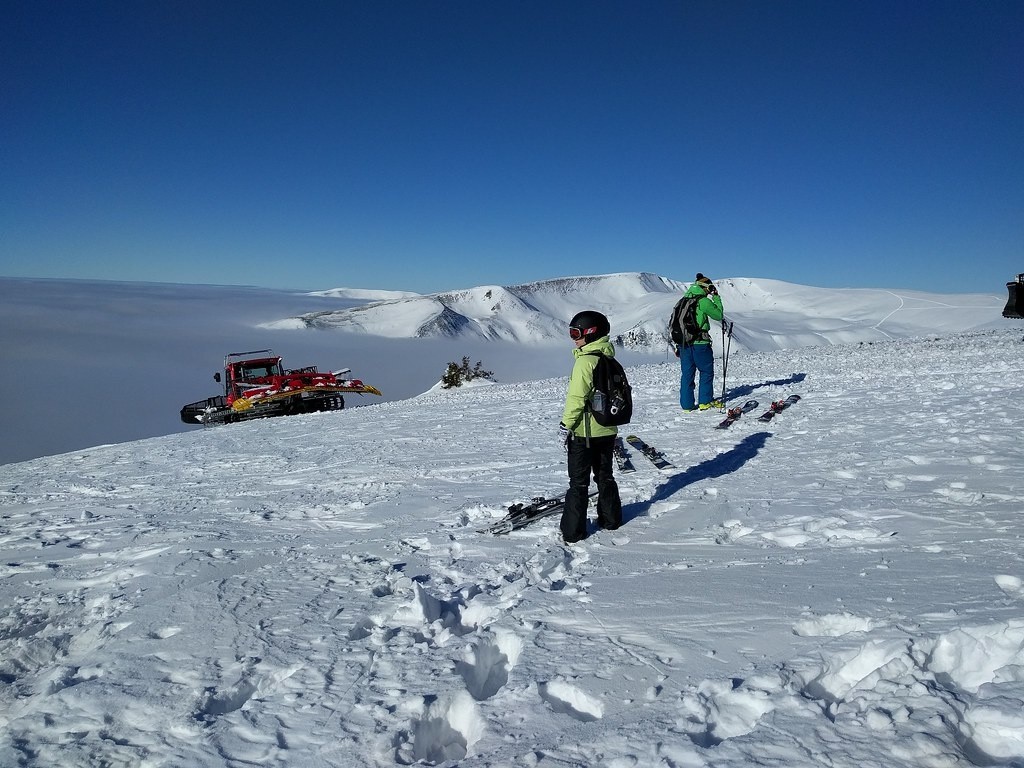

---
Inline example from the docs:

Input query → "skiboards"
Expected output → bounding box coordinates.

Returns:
[613,435,676,475]
[714,393,802,431]
[472,488,598,533]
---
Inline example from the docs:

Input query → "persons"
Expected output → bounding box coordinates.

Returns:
[667,273,724,412]
[553,311,623,542]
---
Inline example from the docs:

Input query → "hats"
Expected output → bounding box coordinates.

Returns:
[696,273,713,292]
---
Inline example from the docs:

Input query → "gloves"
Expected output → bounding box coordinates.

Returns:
[556,426,570,452]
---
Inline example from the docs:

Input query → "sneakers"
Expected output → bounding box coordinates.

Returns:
[684,405,695,412]
[700,401,722,410]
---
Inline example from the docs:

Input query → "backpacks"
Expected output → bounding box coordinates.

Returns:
[583,351,634,448]
[669,296,709,350]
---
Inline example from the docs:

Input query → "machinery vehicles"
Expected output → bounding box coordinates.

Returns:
[180,348,381,427]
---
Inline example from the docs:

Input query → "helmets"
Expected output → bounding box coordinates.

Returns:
[570,310,611,344]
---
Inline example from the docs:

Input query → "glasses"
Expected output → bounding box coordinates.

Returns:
[569,327,582,340]
[707,283,716,293]
